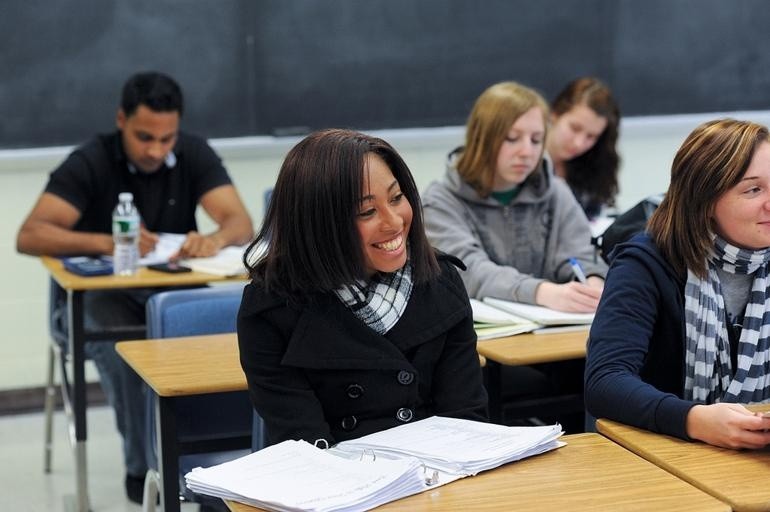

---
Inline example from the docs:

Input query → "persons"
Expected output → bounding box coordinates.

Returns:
[17,69,254,505]
[585,118,770,453]
[544,78,623,224]
[420,82,610,312]
[236,129,490,453]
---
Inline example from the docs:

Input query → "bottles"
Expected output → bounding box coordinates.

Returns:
[112,191,139,282]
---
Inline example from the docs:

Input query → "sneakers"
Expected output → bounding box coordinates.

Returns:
[126,472,160,505]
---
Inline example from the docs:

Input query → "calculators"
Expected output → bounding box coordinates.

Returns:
[66,255,115,276]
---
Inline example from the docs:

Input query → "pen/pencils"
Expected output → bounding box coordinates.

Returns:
[569,259,589,285]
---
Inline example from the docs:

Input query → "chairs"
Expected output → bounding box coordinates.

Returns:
[45,274,82,472]
[250,405,268,451]
[141,288,252,512]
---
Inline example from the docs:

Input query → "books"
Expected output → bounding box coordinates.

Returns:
[483,297,596,326]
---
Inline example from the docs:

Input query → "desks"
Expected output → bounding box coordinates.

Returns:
[42,249,250,442]
[221,431,731,511]
[111,332,248,511]
[476,324,589,432]
[595,400,769,511]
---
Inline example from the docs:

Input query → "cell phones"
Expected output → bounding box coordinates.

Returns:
[148,262,192,273]
